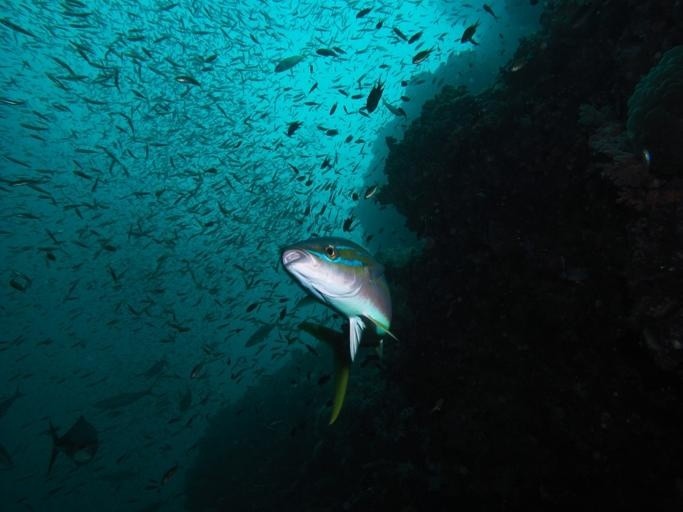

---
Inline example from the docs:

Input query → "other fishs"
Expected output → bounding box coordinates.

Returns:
[0,0,500,512]
